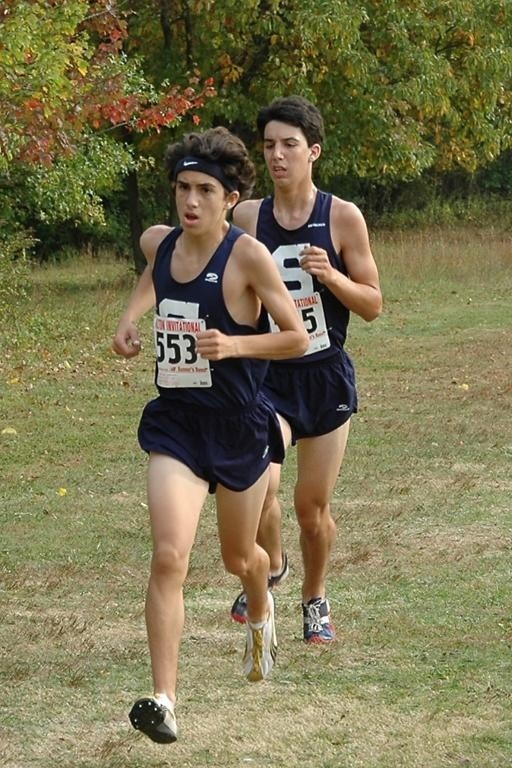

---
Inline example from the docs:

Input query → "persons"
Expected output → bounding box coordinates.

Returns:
[231,96,383,643]
[110,125,307,745]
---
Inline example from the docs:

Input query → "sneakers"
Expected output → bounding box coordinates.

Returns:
[228,548,289,626]
[239,586,279,683]
[301,598,336,645]
[127,692,179,746]
